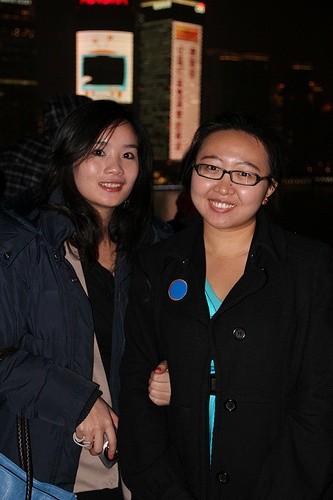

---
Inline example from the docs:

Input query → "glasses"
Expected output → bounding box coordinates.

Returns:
[191,164,270,186]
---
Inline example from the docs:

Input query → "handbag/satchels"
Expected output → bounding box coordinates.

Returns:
[0,416,79,499]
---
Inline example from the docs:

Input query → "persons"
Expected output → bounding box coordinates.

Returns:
[106,105,333,500]
[1,100,173,500]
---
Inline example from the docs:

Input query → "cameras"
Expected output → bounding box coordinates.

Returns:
[92,432,120,469]
